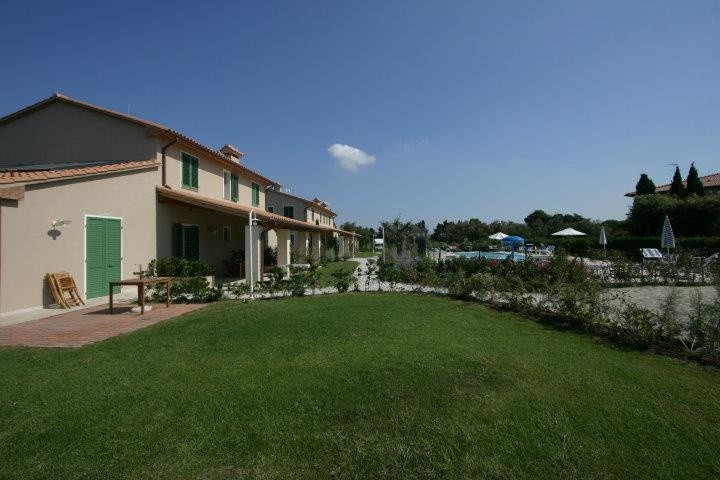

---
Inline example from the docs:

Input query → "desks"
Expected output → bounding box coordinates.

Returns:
[108,278,171,315]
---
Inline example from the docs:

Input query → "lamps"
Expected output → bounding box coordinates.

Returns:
[53,218,72,226]
[208,226,218,233]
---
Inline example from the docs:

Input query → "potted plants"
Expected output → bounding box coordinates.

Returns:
[156,255,216,288]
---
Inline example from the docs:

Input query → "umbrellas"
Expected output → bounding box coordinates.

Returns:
[599,225,608,261]
[661,215,676,257]
[488,232,508,251]
[551,228,589,257]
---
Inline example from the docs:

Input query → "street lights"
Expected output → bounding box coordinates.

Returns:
[249,209,258,302]
[352,230,356,260]
[375,226,385,263]
